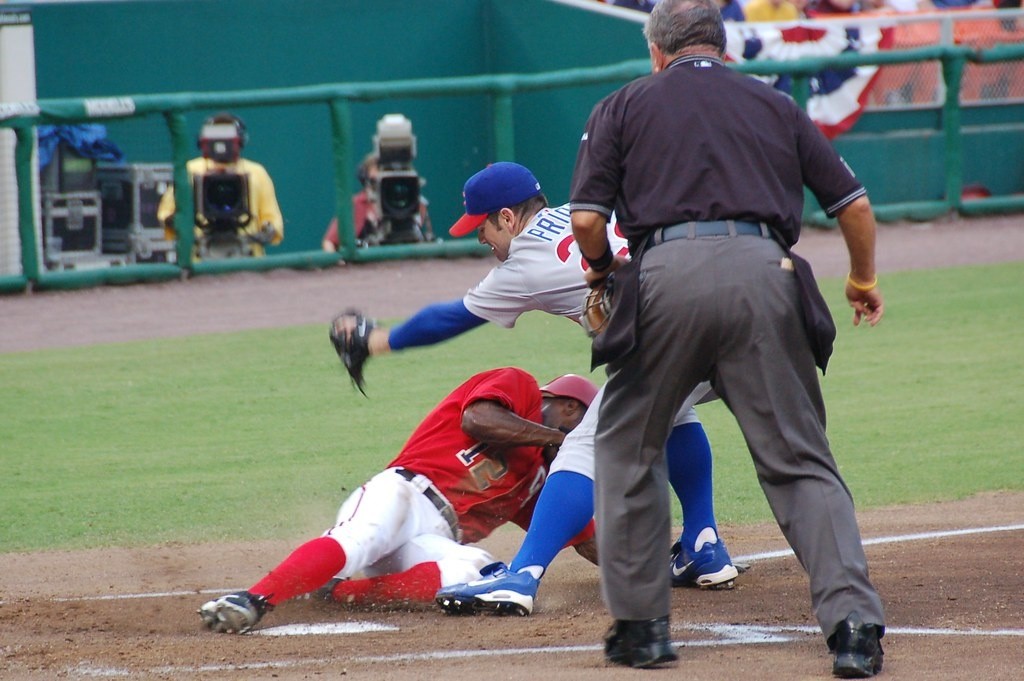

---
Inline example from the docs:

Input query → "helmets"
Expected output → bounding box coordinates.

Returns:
[539,374,599,407]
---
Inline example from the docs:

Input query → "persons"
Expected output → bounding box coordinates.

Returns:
[794,0,868,15]
[327,160,739,617]
[322,153,432,254]
[715,0,744,21]
[156,113,284,262]
[195,367,601,636]
[743,0,800,22]
[567,0,887,678]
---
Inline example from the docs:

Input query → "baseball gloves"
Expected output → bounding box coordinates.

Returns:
[330,304,376,399]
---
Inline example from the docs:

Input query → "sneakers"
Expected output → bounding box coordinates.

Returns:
[196,591,274,635]
[671,537,738,586]
[433,561,541,615]
[290,576,347,603]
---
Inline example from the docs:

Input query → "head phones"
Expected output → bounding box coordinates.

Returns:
[197,116,250,148]
[358,163,368,185]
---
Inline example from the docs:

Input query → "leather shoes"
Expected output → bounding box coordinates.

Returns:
[604,614,678,669]
[828,611,884,677]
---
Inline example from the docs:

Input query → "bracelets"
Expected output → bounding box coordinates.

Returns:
[579,238,613,272]
[351,320,373,348]
[848,271,877,292]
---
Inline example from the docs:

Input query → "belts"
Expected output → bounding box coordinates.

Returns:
[644,219,774,247]
[397,468,460,542]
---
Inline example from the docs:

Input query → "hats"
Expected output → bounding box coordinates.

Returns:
[449,160,543,237]
